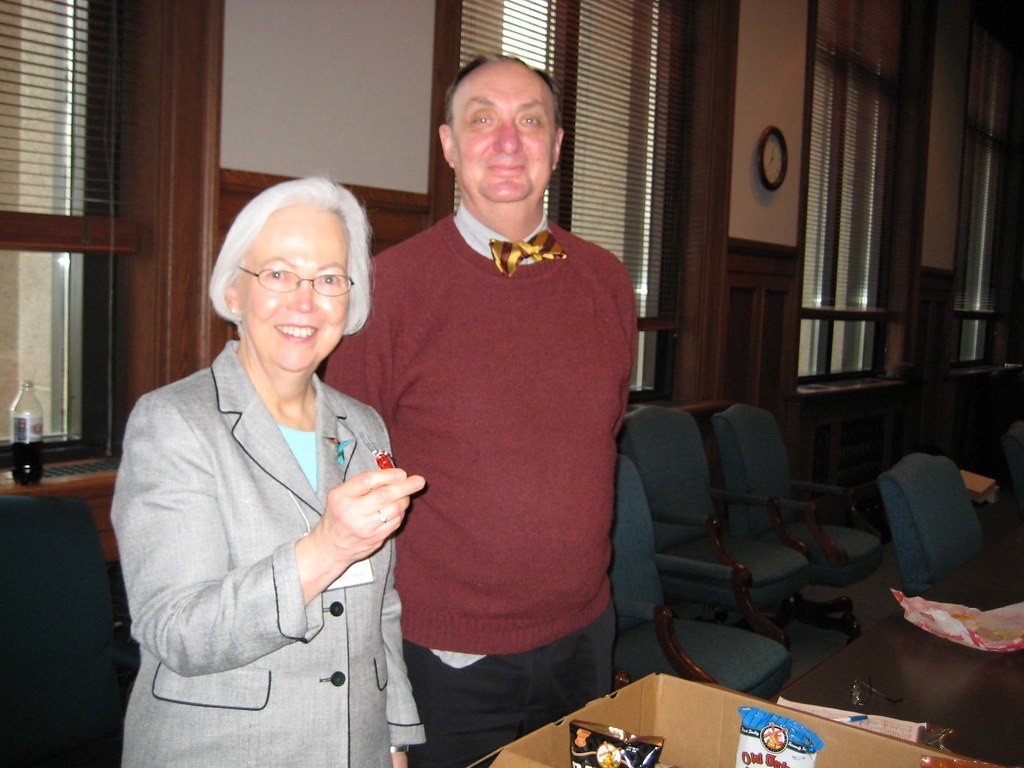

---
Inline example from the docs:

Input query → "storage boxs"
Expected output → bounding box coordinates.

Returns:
[464,673,1011,768]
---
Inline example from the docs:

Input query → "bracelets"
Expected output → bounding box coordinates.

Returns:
[388,743,409,755]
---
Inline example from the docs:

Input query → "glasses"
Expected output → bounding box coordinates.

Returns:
[238,267,355,298]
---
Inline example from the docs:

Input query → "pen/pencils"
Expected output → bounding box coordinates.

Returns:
[364,434,395,469]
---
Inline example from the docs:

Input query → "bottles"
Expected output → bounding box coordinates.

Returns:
[9,380,43,485]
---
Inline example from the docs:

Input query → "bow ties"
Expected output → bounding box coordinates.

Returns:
[490,229,566,276]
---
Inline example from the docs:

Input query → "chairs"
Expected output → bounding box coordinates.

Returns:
[0,492,141,768]
[877,452,982,596]
[616,405,811,635]
[606,453,791,699]
[1001,421,1024,525]
[711,403,883,644]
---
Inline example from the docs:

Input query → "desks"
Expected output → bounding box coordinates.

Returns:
[766,518,1024,768]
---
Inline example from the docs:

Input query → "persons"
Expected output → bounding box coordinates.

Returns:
[323,51,637,768]
[110,175,425,768]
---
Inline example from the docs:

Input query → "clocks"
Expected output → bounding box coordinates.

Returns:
[757,126,788,191]
